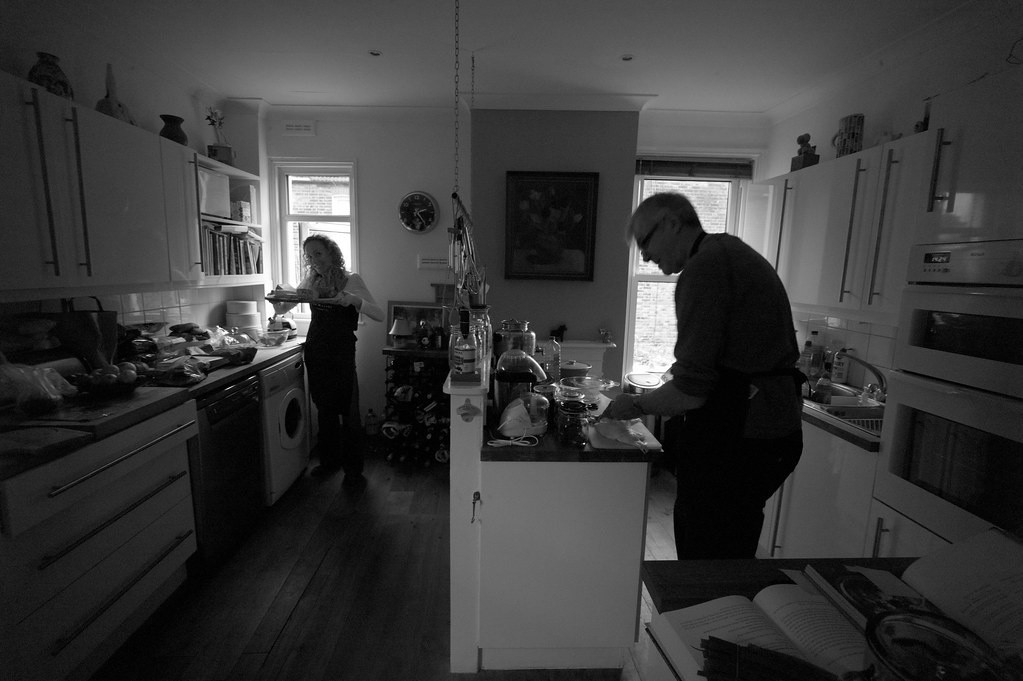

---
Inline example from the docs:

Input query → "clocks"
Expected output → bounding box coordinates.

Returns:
[398,191,440,234]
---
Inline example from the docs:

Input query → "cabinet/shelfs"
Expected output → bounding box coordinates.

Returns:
[1,71,263,303]
[750,62,1023,557]
[0,398,199,681]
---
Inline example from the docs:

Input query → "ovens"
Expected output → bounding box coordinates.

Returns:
[190,375,268,557]
[872,243,1023,544]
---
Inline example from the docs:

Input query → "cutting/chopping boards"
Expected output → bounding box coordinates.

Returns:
[587,421,662,450]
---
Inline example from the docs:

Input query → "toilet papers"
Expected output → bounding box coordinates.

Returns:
[225,313,263,327]
[227,300,257,314]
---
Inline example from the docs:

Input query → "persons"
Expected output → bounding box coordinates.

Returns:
[606,193,803,561]
[266,235,386,495]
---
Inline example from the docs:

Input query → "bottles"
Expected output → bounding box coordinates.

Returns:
[365,408,379,451]
[73,373,94,409]
[824,347,833,373]
[543,337,560,382]
[437,327,444,349]
[814,369,830,404]
[831,347,854,383]
[557,400,589,447]
[384,415,450,467]
[534,385,554,409]
[449,308,491,374]
[795,329,811,383]
[379,410,390,428]
[807,331,824,379]
[495,320,535,361]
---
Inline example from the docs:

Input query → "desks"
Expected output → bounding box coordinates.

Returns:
[640,556,1023,680]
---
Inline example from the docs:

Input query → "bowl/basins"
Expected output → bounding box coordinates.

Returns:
[92,375,147,397]
[558,360,592,383]
[553,377,620,404]
[257,328,290,346]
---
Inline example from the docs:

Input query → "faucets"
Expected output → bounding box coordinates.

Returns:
[835,351,888,397]
[599,328,615,343]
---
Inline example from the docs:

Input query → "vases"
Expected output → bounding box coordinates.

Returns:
[159,113,188,146]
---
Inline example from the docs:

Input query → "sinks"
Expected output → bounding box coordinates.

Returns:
[824,406,887,432]
[799,380,883,406]
[534,339,615,347]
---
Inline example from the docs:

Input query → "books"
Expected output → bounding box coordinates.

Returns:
[809,526,1022,679]
[646,584,878,680]
[643,525,1023,681]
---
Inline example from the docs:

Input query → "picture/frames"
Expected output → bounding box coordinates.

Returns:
[503,168,600,281]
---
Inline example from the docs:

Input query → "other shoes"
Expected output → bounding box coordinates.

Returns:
[342,474,366,496]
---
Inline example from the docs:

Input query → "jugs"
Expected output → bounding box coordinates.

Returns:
[495,350,547,416]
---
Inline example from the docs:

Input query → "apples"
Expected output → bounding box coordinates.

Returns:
[88,362,137,384]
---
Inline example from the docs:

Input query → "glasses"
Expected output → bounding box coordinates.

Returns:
[636,216,664,250]
